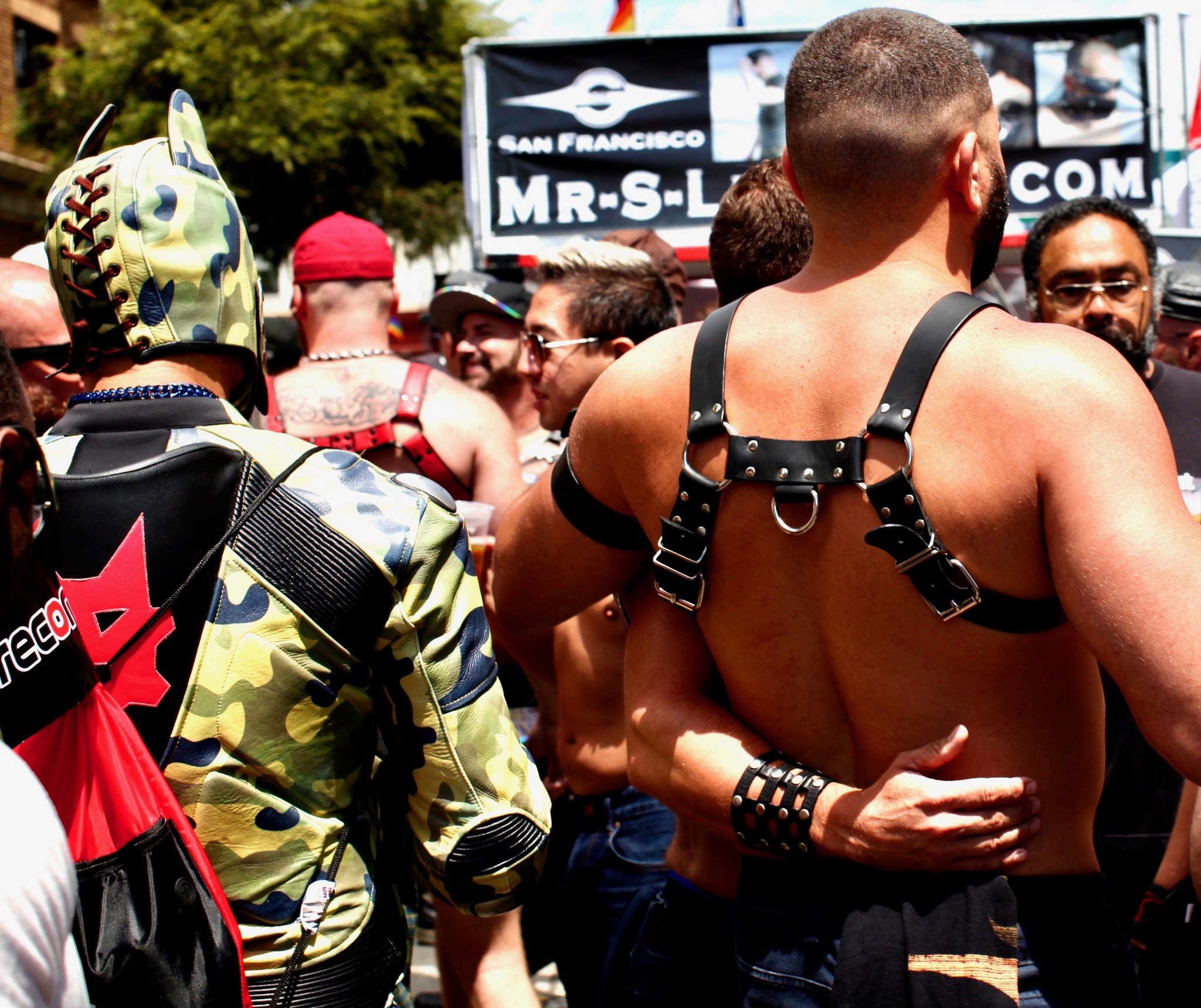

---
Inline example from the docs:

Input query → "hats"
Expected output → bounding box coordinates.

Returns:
[428,281,531,331]
[600,228,687,304]
[1159,274,1201,323]
[44,87,269,423]
[292,210,395,284]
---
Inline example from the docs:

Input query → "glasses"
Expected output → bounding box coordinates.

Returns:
[1074,73,1123,94]
[1037,279,1151,316]
[10,340,76,374]
[519,329,599,365]
[998,101,1032,120]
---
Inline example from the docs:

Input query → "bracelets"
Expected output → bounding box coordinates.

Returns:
[551,446,653,555]
[730,751,831,863]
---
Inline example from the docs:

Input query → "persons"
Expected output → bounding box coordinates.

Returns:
[478,9,1201,1008]
[0,88,674,1008]
[735,46,786,160]
[1035,29,1132,146]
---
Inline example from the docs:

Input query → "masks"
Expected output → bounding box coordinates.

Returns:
[1074,96,1117,119]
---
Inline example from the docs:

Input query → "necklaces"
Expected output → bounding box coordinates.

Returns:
[299,347,393,366]
[66,383,219,409]
